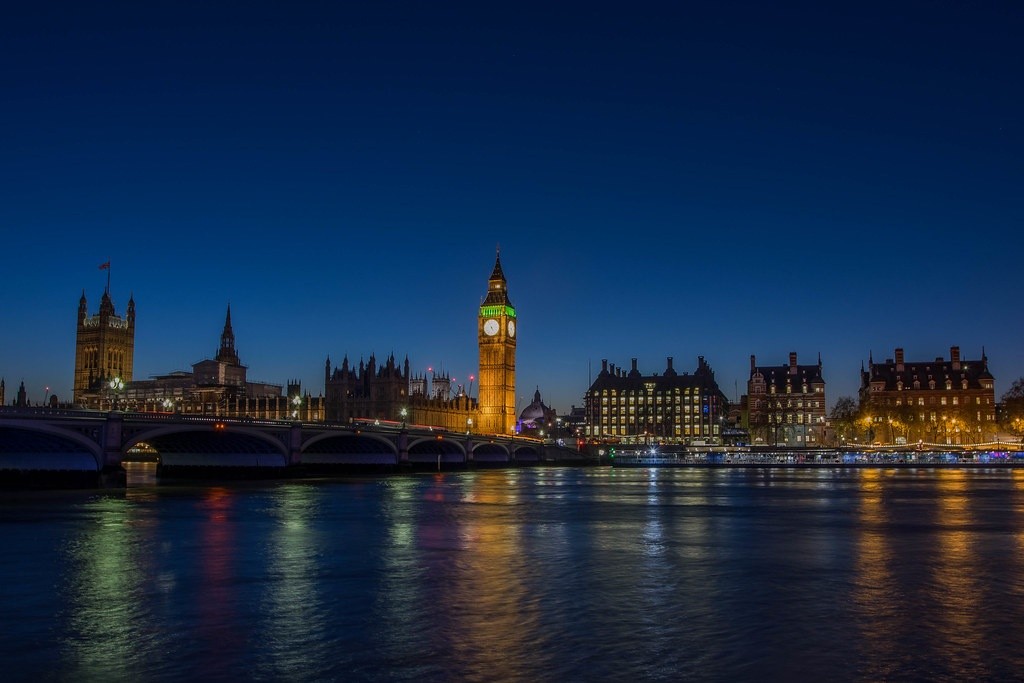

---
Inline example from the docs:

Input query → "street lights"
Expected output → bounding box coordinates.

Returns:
[293,395,301,420]
[511,426,514,441]
[468,419,472,434]
[110,377,124,411]
[401,408,408,428]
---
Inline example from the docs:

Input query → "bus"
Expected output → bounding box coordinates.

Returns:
[682,437,719,446]
